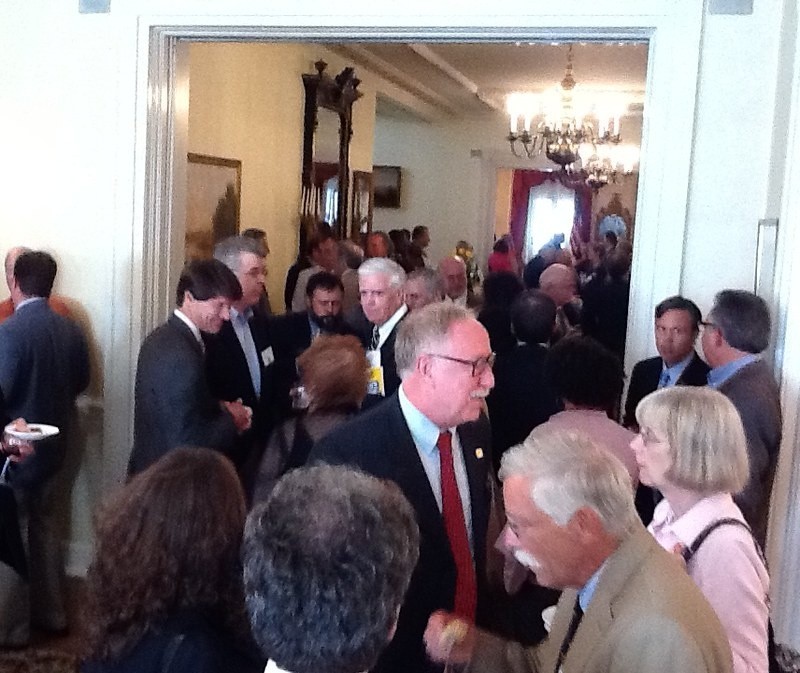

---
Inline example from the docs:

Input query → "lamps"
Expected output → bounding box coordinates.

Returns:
[503,44,641,197]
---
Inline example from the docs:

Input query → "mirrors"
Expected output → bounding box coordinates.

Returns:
[300,56,361,246]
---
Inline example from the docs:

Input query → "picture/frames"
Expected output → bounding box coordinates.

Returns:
[185,148,241,266]
[354,170,373,240]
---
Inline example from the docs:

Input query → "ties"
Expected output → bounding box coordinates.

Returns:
[661,374,671,387]
[368,329,380,352]
[435,432,476,625]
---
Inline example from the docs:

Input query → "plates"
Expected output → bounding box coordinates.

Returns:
[542,605,556,628]
[5,423,59,440]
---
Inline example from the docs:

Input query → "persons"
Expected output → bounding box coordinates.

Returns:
[628,384,769,672]
[239,464,421,673]
[697,290,784,553]
[422,430,735,673]
[1,223,633,673]
[621,296,711,529]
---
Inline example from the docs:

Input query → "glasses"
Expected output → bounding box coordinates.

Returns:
[698,319,713,332]
[641,431,660,448]
[424,351,497,378]
